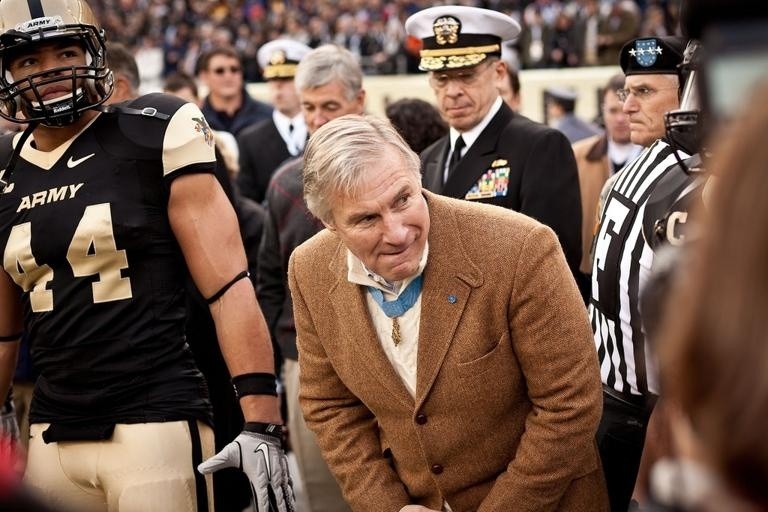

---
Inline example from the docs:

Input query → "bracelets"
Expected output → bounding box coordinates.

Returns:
[232,373,280,400]
[205,270,250,310]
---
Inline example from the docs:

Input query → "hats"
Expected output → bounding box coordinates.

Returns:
[544,87,576,101]
[256,38,314,81]
[404,4,522,72]
[619,35,688,77]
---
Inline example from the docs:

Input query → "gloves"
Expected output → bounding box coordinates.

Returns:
[195,421,299,512]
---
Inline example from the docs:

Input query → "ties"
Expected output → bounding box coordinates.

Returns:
[447,134,467,179]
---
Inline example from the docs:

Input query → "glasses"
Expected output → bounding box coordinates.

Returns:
[615,86,681,101]
[426,61,496,88]
[208,66,242,75]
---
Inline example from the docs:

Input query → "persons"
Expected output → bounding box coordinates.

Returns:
[627,77,766,512]
[194,50,273,139]
[97,0,237,100]
[407,5,583,287]
[254,44,365,512]
[587,37,711,512]
[507,0,681,68]
[238,41,318,201]
[237,2,309,77]
[388,98,446,155]
[543,87,607,144]
[288,114,609,512]
[310,0,407,73]
[570,74,639,298]
[0,0,296,511]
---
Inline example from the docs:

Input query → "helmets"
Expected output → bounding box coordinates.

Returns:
[0,0,115,129]
